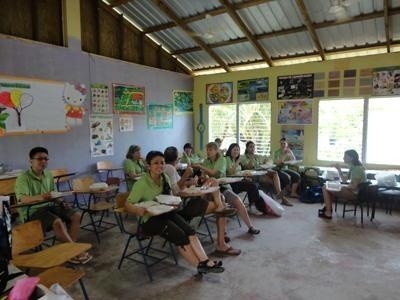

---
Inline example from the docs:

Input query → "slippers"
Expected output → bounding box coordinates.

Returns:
[78,252,93,263]
[68,258,80,265]
[215,208,238,217]
[281,200,294,206]
[275,189,286,199]
[215,246,242,255]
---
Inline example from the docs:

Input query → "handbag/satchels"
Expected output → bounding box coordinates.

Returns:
[300,167,324,190]
[300,184,323,203]
[32,282,74,300]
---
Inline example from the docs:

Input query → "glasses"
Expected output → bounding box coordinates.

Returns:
[30,157,49,161]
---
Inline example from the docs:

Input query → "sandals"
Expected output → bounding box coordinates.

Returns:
[318,211,331,219]
[247,227,261,235]
[223,233,231,241]
[318,207,333,213]
[196,259,224,274]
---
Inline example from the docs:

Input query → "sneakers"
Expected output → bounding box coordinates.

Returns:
[248,208,264,216]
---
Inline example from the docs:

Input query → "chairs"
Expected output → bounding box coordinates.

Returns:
[342,182,373,222]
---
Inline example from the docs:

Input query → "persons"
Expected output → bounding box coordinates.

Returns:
[163,146,241,255]
[273,138,301,198]
[15,147,93,265]
[192,142,260,242]
[319,150,366,219]
[224,143,281,216]
[215,138,225,156]
[241,141,293,206]
[124,151,225,276]
[181,143,199,163]
[124,145,147,191]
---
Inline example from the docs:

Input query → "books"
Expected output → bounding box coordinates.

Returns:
[326,181,342,192]
